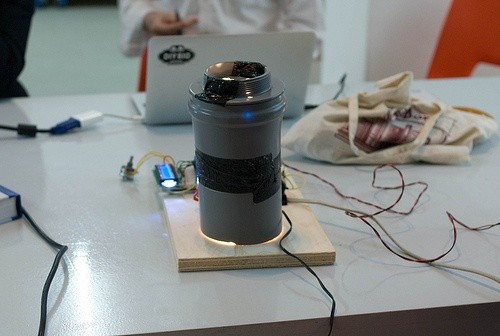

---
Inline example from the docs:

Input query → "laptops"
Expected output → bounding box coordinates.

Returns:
[134,31,317,124]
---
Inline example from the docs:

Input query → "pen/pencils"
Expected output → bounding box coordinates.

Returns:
[176,12,181,35]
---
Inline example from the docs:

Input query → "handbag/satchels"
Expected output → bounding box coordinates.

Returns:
[276,67,498,169]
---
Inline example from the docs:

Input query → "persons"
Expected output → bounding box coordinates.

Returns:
[118,0,327,92]
[0,0,36,99]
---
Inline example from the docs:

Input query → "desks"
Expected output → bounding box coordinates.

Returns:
[0,75,500,336]
